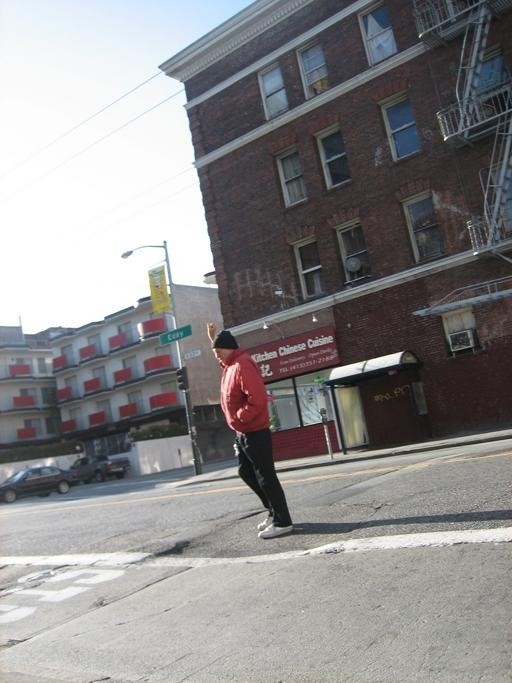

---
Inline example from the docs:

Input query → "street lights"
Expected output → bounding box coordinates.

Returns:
[119,237,205,478]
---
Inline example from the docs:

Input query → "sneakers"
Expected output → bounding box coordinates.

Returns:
[258,522,294,539]
[257,516,275,531]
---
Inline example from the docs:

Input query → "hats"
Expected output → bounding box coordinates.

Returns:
[211,330,238,350]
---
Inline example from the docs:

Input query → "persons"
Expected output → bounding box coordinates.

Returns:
[206,321,294,539]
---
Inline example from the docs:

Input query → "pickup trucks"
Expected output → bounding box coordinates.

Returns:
[66,452,132,485]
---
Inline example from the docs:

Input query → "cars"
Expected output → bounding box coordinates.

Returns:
[0,463,80,503]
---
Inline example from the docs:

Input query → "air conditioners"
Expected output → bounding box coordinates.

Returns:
[447,329,479,352]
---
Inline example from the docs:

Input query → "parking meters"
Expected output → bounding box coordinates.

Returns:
[318,406,334,461]
